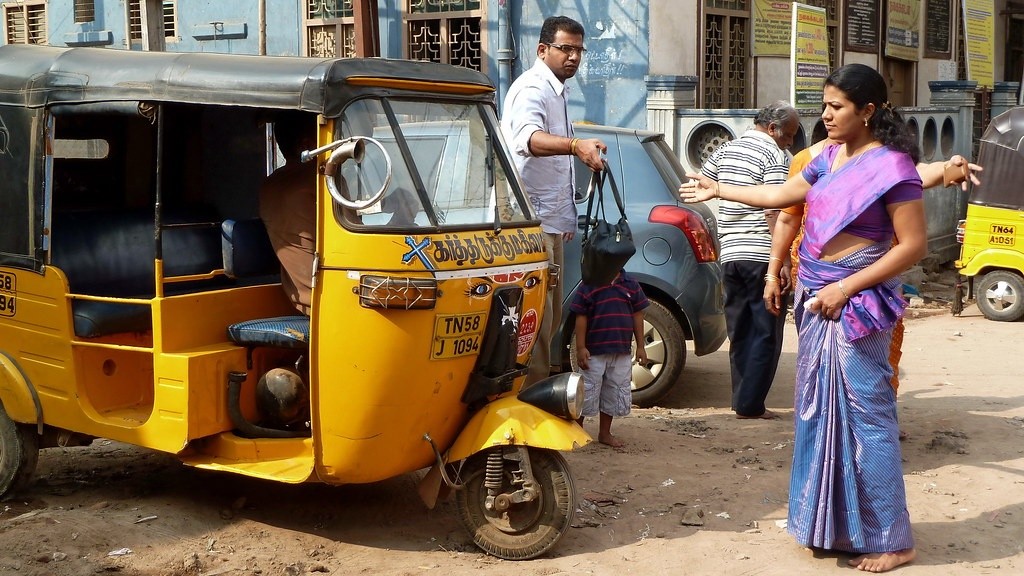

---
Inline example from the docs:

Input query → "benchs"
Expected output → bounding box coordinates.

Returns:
[231,311,312,439]
[36,207,262,413]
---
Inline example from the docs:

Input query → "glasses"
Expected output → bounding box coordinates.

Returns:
[544,42,588,56]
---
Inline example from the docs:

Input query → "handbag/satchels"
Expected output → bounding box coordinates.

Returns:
[579,159,635,290]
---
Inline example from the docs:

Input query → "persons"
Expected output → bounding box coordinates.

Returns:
[679,63,927,573]
[500,15,608,396]
[258,110,365,323]
[570,267,650,447]
[688,101,800,419]
[762,97,983,438]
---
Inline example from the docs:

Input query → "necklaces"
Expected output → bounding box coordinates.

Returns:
[826,137,874,187]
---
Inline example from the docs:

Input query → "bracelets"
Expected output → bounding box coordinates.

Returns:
[769,257,784,267]
[568,138,580,156]
[716,182,720,200]
[764,274,780,286]
[838,279,850,300]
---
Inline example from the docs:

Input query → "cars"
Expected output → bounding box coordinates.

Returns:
[357,122,731,407]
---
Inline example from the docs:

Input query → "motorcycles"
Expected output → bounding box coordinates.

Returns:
[951,106,1024,322]
[0,44,592,561]
